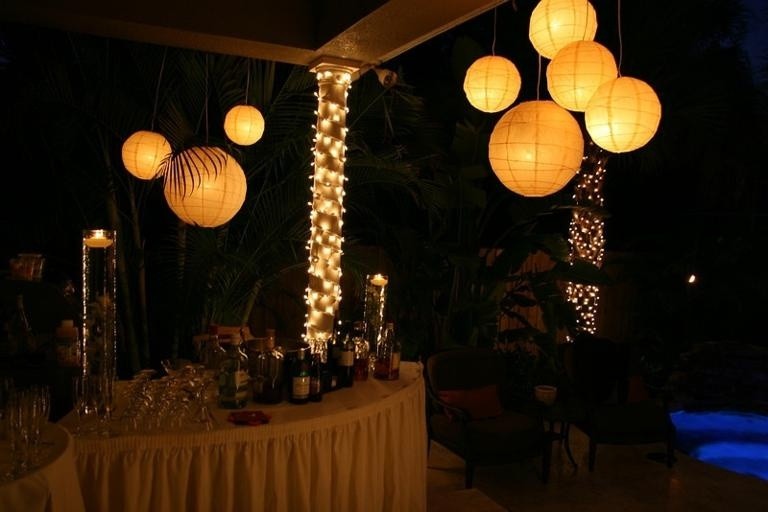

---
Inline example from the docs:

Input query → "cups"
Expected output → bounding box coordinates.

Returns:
[108,364,213,433]
[1,383,52,476]
[69,375,108,442]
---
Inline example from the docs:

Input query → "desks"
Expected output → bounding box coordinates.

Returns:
[510,390,596,475]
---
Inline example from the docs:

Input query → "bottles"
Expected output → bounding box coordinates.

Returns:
[55,318,80,368]
[328,317,401,385]
[203,321,249,409]
[291,348,324,404]
[254,330,285,404]
[12,294,35,337]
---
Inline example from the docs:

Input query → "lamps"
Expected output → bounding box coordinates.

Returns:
[160,49,250,229]
[222,54,269,151]
[118,44,172,185]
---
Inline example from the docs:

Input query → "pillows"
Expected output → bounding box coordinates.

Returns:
[435,383,507,422]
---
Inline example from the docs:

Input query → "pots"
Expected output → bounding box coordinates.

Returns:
[237,336,313,400]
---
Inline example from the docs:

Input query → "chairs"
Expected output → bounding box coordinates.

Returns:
[420,339,553,494]
